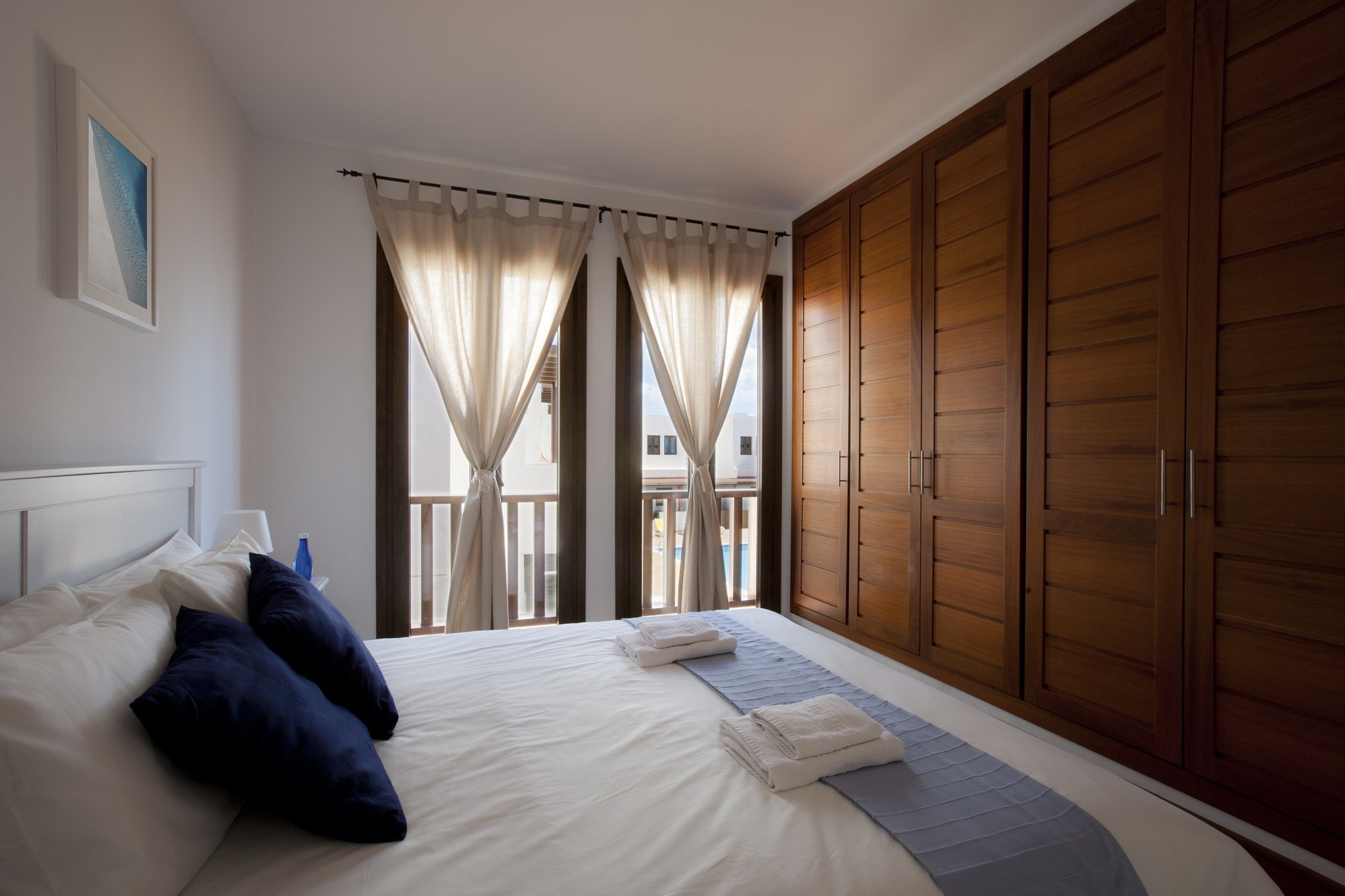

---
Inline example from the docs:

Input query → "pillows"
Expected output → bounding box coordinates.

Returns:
[247,552,398,743]
[128,606,407,844]
[159,532,266,622]
[0,581,238,896]
[72,531,203,611]
[0,582,85,652]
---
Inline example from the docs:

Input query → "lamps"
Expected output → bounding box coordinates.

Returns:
[219,510,273,553]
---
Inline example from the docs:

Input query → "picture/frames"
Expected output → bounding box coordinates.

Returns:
[54,63,159,334]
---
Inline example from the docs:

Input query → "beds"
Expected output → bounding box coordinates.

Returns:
[1,462,1285,896]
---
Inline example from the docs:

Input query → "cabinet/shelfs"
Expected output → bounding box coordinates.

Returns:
[853,92,1025,700]
[793,194,853,625]
[1024,0,1345,844]
